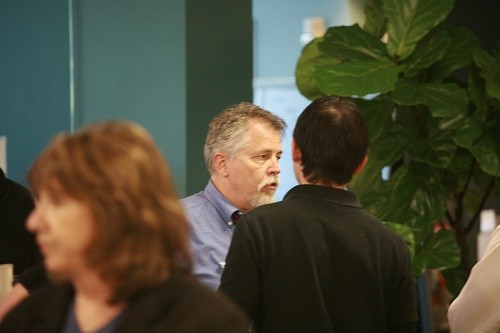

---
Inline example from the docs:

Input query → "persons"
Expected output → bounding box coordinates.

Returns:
[218,94,431,332]
[448,214,499,331]
[172,102,287,294]
[0,118,253,333]
